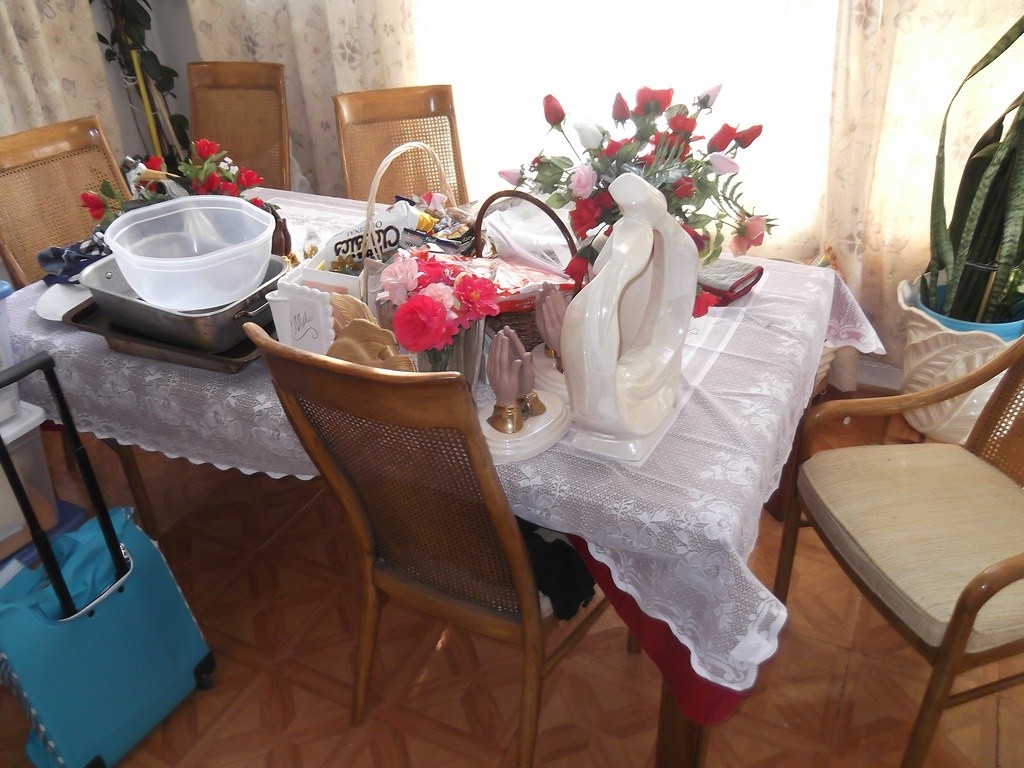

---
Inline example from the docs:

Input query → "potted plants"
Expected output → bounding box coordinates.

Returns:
[896,14,1024,446]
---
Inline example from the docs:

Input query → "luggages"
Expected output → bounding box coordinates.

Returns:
[0,350,217,768]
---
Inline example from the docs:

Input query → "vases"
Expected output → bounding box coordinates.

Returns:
[587,225,613,282]
[416,325,465,377]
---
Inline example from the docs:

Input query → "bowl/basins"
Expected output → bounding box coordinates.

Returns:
[104,195,276,313]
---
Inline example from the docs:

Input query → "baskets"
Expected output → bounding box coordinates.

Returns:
[466,190,588,349]
[361,142,492,269]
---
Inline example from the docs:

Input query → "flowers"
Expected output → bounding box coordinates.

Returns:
[374,245,500,371]
[78,137,266,219]
[497,84,780,290]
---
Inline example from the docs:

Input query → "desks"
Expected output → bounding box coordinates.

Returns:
[7,187,887,768]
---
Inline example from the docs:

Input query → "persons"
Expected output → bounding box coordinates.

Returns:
[535,281,574,375]
[486,326,547,434]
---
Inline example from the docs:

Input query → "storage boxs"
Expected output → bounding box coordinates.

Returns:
[0,501,88,571]
[0,280,20,422]
[0,400,60,562]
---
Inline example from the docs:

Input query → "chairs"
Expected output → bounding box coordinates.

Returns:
[184,61,290,189]
[331,84,470,205]
[772,333,1024,768]
[0,115,136,292]
[240,321,643,768]
